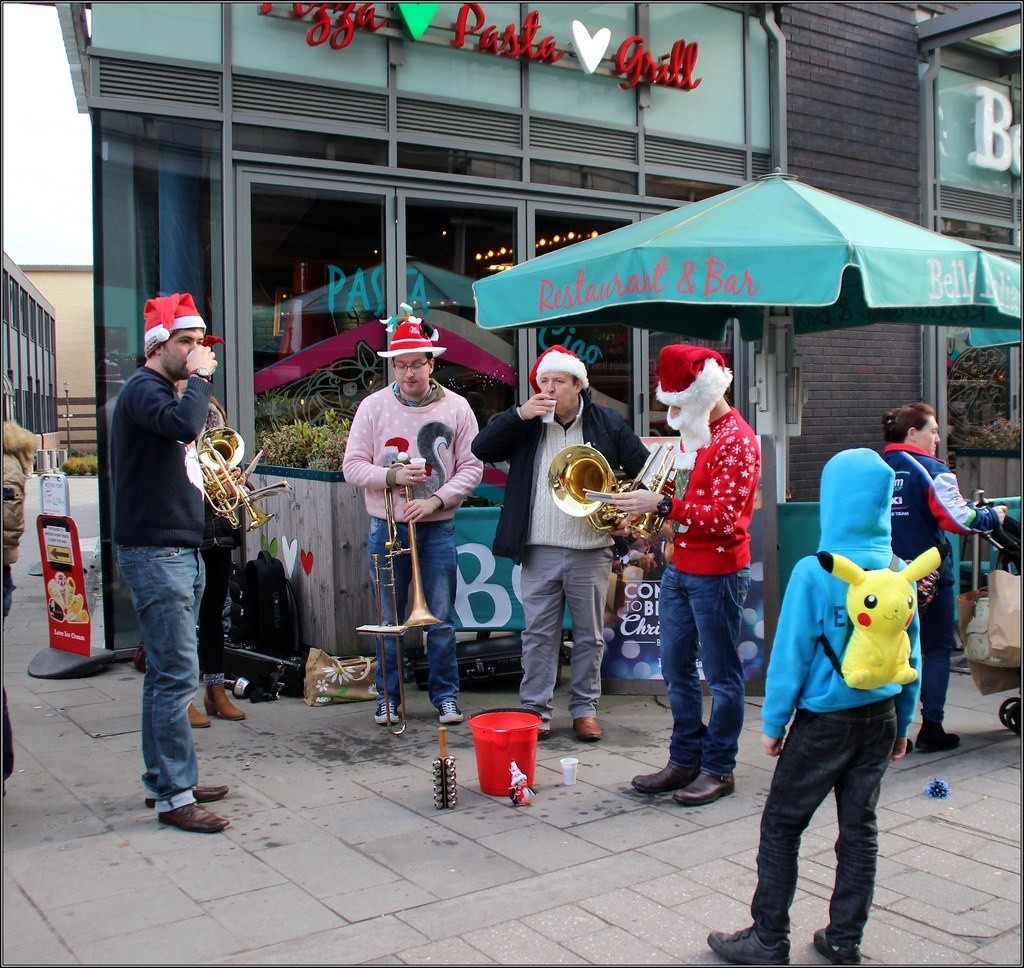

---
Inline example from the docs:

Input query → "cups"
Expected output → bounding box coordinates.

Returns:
[540,401,557,423]
[560,758,579,785]
[410,458,426,477]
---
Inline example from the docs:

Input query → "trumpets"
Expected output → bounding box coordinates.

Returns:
[626,444,678,543]
[191,436,274,535]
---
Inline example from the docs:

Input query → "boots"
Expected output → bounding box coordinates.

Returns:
[204,685,246,720]
[188,703,211,728]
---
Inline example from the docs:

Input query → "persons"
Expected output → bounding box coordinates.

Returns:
[108,291,232,833]
[608,342,764,807]
[705,444,927,968]
[2,414,37,805]
[171,379,255,730]
[340,302,485,727]
[471,343,653,743]
[879,401,1008,755]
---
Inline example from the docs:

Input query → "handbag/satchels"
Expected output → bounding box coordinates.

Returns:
[956,568,1021,697]
[303,647,379,708]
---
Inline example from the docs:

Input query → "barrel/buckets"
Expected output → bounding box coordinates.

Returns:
[467,707,542,796]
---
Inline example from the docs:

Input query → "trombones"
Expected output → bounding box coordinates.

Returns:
[371,463,443,737]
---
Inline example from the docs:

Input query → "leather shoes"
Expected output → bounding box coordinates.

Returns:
[573,717,602,741]
[631,761,701,794]
[537,728,552,741]
[145,785,229,808]
[672,770,736,806]
[158,801,230,833]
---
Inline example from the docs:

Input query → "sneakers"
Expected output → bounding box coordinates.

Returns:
[375,702,400,724]
[814,928,862,964]
[707,926,790,965]
[438,701,464,724]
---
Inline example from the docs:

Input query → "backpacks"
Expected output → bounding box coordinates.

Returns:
[229,549,303,698]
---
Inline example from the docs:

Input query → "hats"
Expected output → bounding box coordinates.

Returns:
[377,301,447,359]
[144,293,207,343]
[655,344,734,454]
[529,345,589,400]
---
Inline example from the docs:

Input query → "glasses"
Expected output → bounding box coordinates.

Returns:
[392,359,431,374]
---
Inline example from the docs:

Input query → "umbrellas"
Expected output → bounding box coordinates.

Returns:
[248,251,477,318]
[470,165,1023,354]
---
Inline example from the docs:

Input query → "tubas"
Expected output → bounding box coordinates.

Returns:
[546,442,629,533]
[196,426,246,471]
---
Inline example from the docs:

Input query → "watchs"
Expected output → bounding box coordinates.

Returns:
[187,367,212,383]
[655,495,673,518]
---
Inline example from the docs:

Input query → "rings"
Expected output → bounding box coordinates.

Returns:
[410,476,414,481]
[626,505,630,511]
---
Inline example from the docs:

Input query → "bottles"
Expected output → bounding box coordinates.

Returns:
[432,727,458,810]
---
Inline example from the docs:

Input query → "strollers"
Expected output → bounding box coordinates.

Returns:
[972,493,1022,736]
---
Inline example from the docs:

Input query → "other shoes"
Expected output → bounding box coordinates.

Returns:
[905,738,913,754]
[915,718,960,752]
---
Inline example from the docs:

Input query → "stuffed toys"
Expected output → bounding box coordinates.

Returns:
[816,543,950,690]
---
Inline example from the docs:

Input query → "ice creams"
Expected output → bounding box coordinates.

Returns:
[47,572,89,624]
